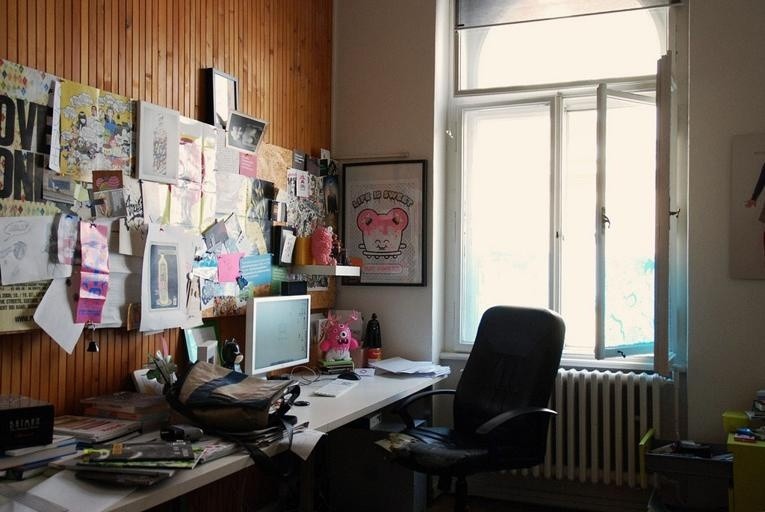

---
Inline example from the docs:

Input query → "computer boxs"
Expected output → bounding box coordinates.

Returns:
[325,416,427,512]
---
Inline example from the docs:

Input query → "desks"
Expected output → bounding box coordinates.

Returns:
[0,372,447,512]
[721,410,764,511]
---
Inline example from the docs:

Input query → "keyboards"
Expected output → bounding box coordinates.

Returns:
[313,378,358,397]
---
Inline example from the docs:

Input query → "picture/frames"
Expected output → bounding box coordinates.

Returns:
[727,132,765,283]
[340,158,429,287]
[207,66,238,128]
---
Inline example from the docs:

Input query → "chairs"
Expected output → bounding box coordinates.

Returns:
[383,304,567,512]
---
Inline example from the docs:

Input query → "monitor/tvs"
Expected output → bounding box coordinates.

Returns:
[245,294,311,386]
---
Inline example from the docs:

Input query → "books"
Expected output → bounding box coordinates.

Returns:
[1,387,310,490]
[317,358,354,376]
[747,388,765,420]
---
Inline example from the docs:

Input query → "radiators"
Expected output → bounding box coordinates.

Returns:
[501,368,678,489]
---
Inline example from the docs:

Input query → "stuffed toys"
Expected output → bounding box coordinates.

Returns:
[318,309,359,362]
[311,225,338,266]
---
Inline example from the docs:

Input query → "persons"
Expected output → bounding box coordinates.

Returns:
[365,312,383,348]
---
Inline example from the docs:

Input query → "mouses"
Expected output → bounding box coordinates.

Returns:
[338,372,361,380]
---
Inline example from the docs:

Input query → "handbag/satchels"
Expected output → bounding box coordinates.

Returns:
[166,359,300,435]
